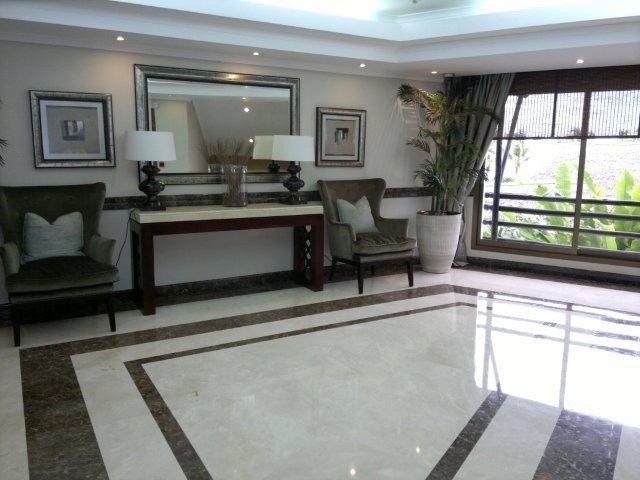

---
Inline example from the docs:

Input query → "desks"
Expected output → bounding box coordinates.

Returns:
[129,200,325,317]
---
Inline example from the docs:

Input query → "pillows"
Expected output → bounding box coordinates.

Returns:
[336,193,379,236]
[20,209,86,264]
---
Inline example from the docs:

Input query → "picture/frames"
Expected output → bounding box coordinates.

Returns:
[315,107,367,168]
[28,88,117,170]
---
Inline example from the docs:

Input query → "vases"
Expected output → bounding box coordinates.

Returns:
[223,165,249,208]
[207,163,226,174]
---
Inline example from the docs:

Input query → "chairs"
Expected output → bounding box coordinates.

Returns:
[317,178,417,294]
[0,181,120,347]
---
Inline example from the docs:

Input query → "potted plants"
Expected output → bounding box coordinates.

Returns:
[396,82,502,275]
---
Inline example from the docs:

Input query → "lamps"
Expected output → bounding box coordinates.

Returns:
[122,129,177,212]
[271,134,316,205]
[252,134,280,174]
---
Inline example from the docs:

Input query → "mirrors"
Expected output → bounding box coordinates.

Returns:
[133,62,300,186]
